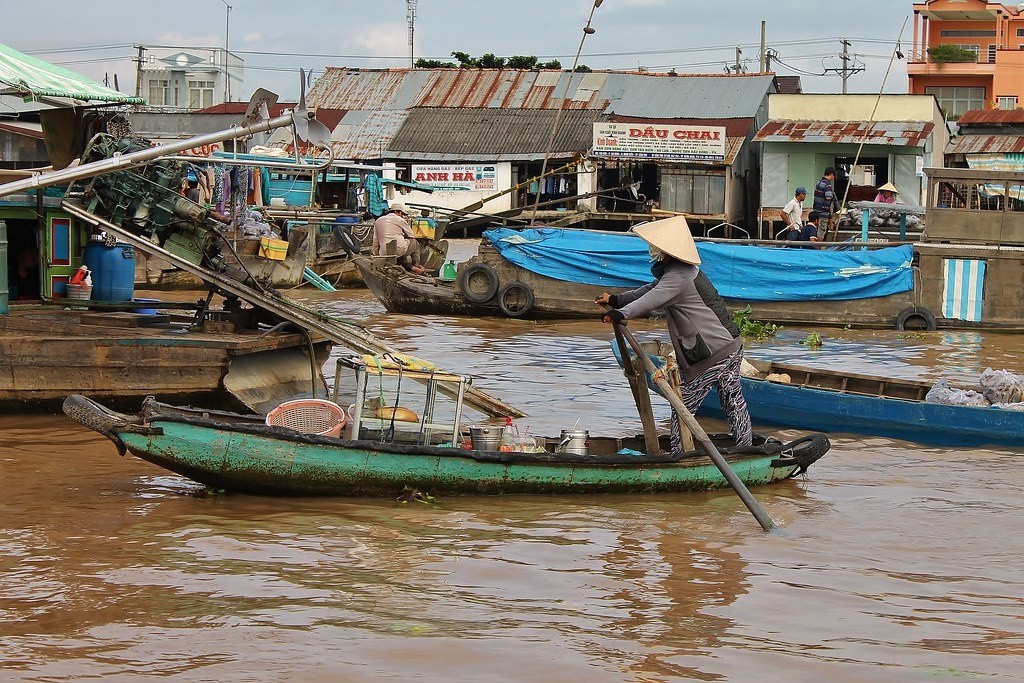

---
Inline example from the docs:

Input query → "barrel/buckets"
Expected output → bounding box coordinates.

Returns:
[133,298,161,315]
[65,284,93,310]
[556,429,590,457]
[462,413,506,451]
[336,211,360,228]
[82,239,136,304]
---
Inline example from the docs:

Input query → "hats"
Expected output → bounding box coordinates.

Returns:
[877,183,899,193]
[796,187,809,194]
[632,215,701,265]
[391,204,408,214]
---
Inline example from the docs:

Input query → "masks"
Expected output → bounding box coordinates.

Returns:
[649,246,665,261]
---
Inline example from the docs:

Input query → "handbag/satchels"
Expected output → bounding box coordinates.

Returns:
[833,194,840,214]
[829,198,834,217]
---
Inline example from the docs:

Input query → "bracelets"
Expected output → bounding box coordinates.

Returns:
[788,223,791,226]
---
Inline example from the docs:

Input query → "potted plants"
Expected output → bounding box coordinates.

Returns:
[925,44,977,70]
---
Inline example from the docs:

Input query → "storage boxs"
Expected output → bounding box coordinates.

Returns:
[409,221,436,240]
[258,237,289,260]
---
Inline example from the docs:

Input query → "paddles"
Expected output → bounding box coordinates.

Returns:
[596,295,781,531]
[604,312,641,418]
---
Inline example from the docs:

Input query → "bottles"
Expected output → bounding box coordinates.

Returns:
[69,264,87,285]
[502,416,514,445]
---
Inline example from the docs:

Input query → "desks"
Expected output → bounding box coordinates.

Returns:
[332,357,467,446]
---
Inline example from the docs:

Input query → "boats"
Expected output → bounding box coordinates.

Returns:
[358,230,1024,332]
[610,334,1024,448]
[59,394,831,491]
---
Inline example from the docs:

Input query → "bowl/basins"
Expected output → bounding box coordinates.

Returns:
[556,208,567,211]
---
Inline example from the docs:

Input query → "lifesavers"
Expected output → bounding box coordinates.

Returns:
[460,264,499,304]
[782,432,832,471]
[895,305,938,332]
[500,280,534,317]
[333,224,360,254]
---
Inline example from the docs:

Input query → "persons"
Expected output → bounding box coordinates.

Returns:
[187,181,199,203]
[8,249,37,297]
[594,215,752,455]
[372,204,426,275]
[779,167,838,250]
[874,182,900,204]
